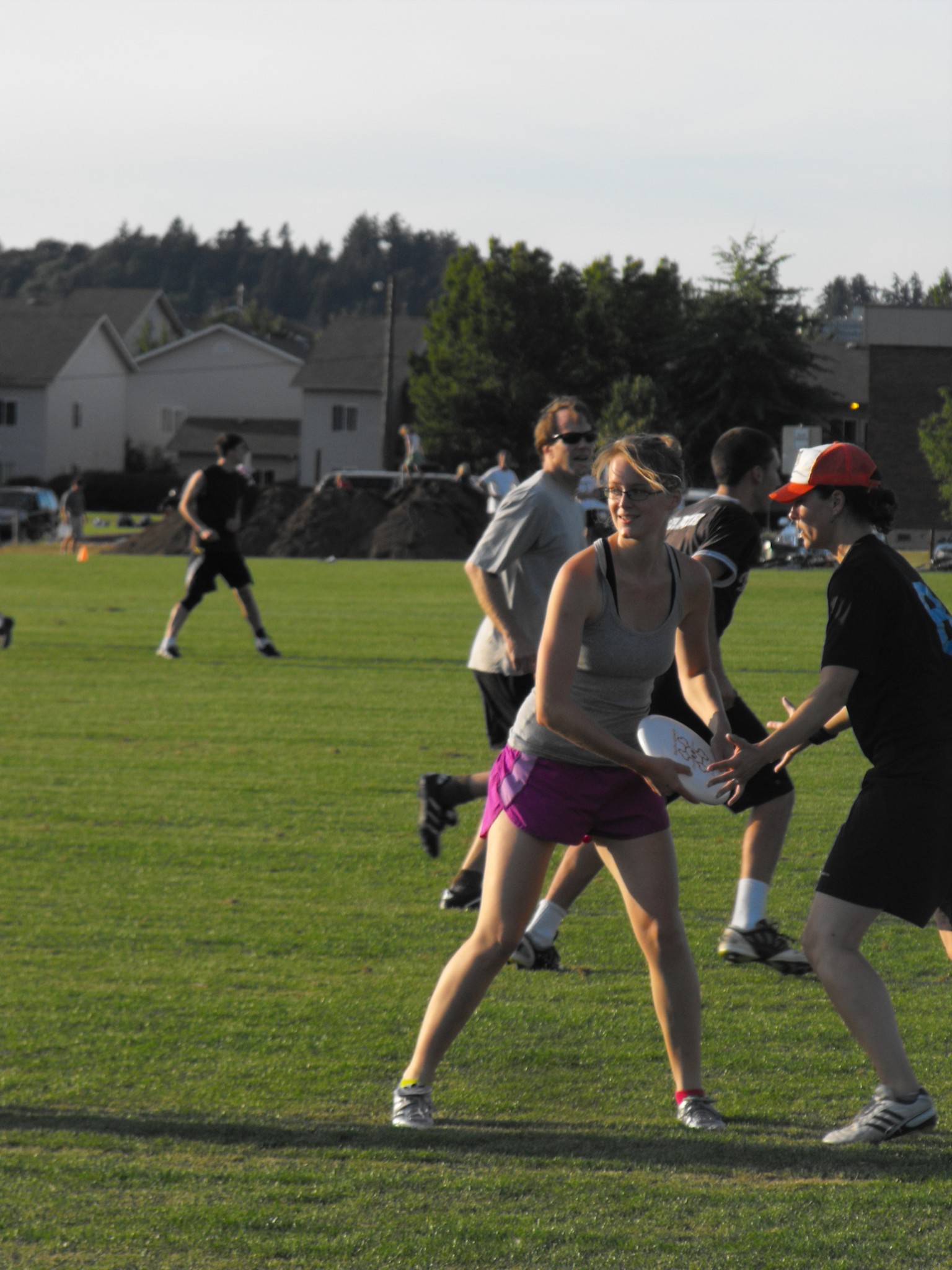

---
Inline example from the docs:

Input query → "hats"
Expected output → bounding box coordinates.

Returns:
[768,440,881,504]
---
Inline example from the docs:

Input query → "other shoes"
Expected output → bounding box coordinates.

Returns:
[255,639,280,658]
[156,645,181,660]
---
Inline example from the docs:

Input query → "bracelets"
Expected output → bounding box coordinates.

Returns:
[809,726,837,745]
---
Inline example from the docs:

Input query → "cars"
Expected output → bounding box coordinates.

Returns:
[0,485,59,544]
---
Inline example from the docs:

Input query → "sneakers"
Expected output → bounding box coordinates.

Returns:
[717,919,814,976]
[438,888,480,912]
[507,933,562,971]
[417,773,458,859]
[391,1086,434,1131]
[677,1095,726,1133]
[820,1085,938,1145]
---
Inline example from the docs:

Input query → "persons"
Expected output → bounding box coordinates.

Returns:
[415,398,598,913]
[705,443,951,1144]
[578,468,613,536]
[397,423,427,474]
[508,427,812,974]
[390,433,731,1135]
[155,433,285,660]
[452,451,522,521]
[59,478,89,555]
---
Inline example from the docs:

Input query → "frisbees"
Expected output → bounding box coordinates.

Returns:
[636,713,733,807]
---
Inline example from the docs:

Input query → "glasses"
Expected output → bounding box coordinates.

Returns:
[544,431,597,444]
[603,487,675,501]
